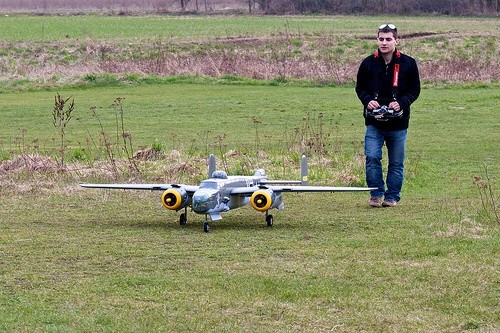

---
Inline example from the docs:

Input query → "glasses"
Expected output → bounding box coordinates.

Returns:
[379,23,397,31]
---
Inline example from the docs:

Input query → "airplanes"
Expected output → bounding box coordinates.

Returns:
[78,154,378,232]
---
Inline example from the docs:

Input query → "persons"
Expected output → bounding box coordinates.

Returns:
[355,24,421,208]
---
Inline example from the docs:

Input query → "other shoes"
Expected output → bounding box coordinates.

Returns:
[370,197,381,206]
[382,198,397,207]
